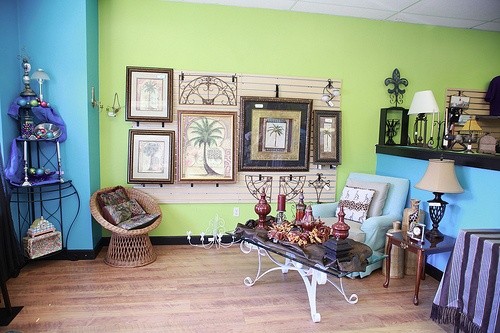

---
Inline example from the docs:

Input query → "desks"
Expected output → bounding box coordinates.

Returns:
[429,229,500,333]
[383,229,456,306]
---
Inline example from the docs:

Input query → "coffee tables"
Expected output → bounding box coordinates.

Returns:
[225,227,389,324]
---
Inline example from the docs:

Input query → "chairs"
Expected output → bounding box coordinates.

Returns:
[90,186,162,267]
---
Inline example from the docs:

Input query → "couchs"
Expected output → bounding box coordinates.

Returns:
[312,173,409,279]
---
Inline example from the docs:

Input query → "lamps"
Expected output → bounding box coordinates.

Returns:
[31,69,50,103]
[462,119,482,154]
[414,154,462,242]
[406,90,440,147]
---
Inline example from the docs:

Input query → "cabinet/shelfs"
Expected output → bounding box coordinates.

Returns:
[10,138,81,269]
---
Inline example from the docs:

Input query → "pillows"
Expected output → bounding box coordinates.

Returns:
[98,185,160,230]
[353,181,390,217]
[334,185,376,224]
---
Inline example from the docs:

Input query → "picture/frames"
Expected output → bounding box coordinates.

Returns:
[313,110,342,166]
[124,66,174,123]
[237,96,313,172]
[177,110,236,184]
[127,128,174,185]
